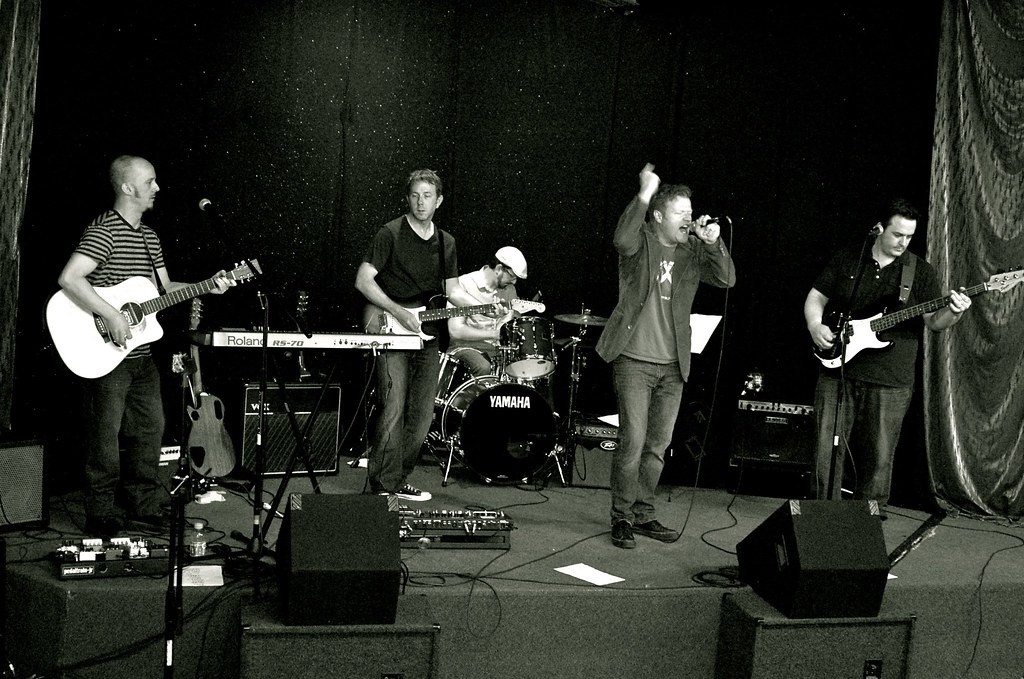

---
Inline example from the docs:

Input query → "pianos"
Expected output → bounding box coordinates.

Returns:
[189,324,425,552]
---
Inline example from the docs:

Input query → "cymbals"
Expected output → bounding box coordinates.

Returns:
[559,311,609,327]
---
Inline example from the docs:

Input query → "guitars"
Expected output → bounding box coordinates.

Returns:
[363,298,547,343]
[813,266,1024,370]
[44,257,265,380]
[181,295,238,479]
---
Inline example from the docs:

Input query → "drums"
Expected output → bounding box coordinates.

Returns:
[434,350,474,408]
[500,315,558,381]
[440,375,559,486]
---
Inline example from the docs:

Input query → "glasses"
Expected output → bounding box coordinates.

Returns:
[503,268,517,280]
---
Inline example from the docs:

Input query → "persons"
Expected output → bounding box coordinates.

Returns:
[448,246,527,377]
[353,170,509,501]
[59,156,237,536]
[595,163,735,550]
[804,203,972,518]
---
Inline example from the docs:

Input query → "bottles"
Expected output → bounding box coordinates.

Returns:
[189,523,205,558]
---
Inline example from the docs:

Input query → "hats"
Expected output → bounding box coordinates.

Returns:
[496,246,527,278]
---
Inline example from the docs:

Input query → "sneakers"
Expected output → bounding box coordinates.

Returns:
[86,514,128,537]
[631,519,677,538]
[395,482,432,502]
[611,521,636,548]
[124,504,172,527]
